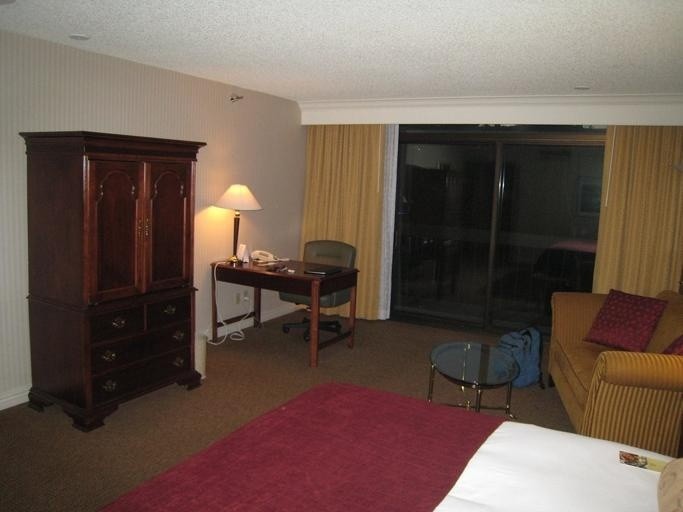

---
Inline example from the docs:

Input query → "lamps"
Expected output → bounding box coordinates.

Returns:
[209,184,263,267]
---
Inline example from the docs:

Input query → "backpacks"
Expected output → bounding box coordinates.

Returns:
[494,325,546,391]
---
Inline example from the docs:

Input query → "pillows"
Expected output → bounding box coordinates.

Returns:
[582,289,668,353]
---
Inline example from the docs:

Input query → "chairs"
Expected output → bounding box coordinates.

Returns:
[279,239,356,344]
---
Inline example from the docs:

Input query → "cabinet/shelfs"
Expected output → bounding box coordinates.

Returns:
[25,287,201,433]
[18,130,209,310]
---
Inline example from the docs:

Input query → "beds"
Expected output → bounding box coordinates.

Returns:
[86,380,675,512]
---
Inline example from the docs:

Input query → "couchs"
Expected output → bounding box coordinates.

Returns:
[546,288,683,459]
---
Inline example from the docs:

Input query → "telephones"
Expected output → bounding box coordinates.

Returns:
[251,250,290,262]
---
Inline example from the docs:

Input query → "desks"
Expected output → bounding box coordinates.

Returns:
[210,250,360,368]
